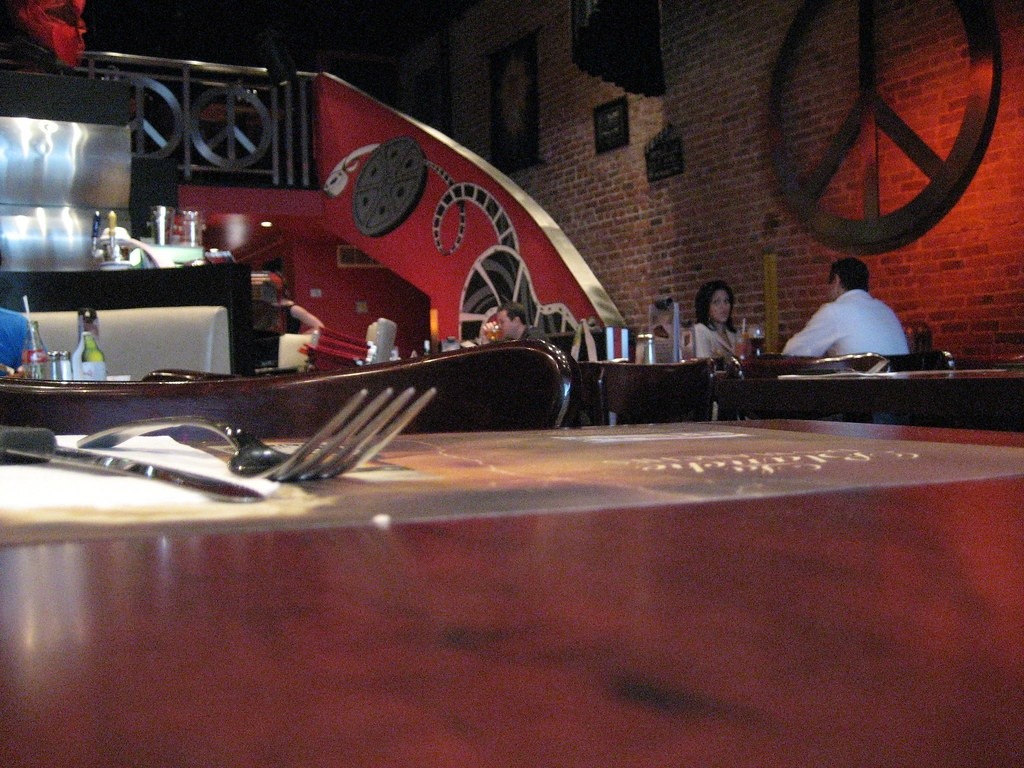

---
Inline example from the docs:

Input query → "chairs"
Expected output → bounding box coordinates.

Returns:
[0,326,1024,768]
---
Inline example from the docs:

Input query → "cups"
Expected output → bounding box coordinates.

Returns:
[150,205,174,246]
[180,209,204,247]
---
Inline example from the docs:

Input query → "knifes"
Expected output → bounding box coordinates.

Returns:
[2,429,266,504]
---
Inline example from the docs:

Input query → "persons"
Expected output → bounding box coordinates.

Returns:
[498,303,548,342]
[0,308,49,381]
[692,280,744,359]
[777,257,912,358]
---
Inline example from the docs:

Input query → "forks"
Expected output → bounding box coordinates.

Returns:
[77,382,442,484]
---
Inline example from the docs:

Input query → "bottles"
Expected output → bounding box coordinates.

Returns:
[80,335,107,382]
[635,334,645,363]
[422,340,431,355]
[733,329,749,358]
[645,334,658,364]
[21,321,49,383]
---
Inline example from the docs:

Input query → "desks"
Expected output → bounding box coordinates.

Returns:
[780,364,1024,434]
[0,417,1024,768]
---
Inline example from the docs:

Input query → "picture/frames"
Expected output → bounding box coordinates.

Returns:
[593,96,629,155]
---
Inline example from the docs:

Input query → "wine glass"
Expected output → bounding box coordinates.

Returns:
[745,323,765,356]
